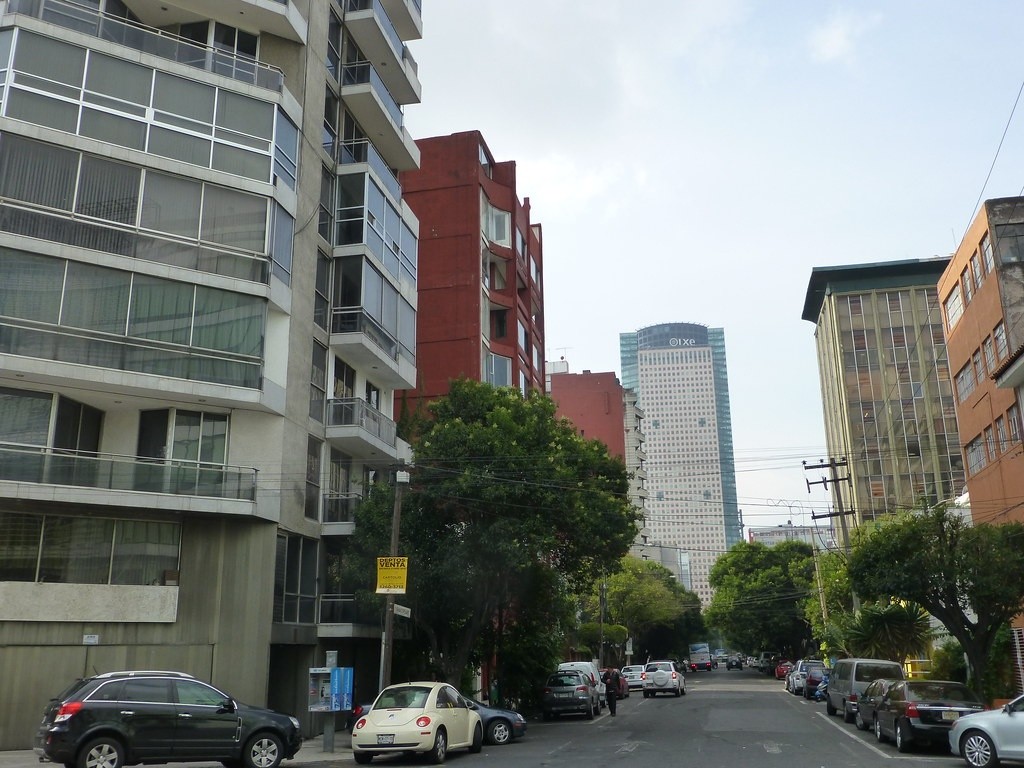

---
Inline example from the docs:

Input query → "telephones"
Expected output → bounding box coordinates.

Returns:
[321,684,330,698]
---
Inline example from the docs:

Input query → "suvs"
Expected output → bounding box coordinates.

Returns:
[642,660,686,698]
[34,671,302,768]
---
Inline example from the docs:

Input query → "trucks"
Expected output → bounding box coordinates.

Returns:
[688,643,711,671]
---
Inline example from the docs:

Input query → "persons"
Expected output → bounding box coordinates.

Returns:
[601,665,623,717]
[710,655,715,667]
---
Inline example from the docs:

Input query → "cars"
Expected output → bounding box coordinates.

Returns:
[543,670,601,720]
[949,695,1024,768]
[558,662,646,708]
[712,649,781,672]
[462,695,527,746]
[775,659,985,753]
[352,681,483,762]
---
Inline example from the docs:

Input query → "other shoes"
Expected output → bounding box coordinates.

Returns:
[611,713,616,716]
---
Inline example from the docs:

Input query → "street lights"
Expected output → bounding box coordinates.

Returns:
[772,528,828,633]
